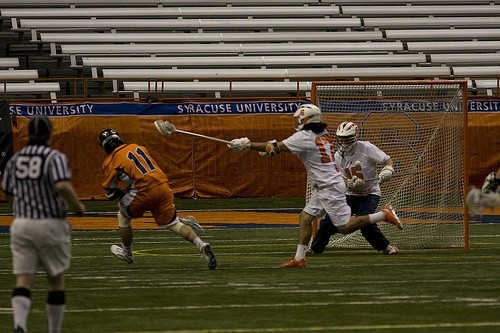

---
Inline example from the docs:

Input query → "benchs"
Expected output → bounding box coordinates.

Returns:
[0,0,500,105]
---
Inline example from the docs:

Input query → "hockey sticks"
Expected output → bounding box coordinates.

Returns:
[465,188,500,209]
[178,215,205,234]
[153,120,232,144]
[342,168,433,193]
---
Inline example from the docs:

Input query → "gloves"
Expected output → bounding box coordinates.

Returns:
[259,141,281,157]
[483,173,500,193]
[227,137,251,152]
[378,165,396,183]
[347,175,365,190]
[104,188,121,201]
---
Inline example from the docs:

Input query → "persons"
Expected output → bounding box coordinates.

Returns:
[466,172,500,208]
[97,127,217,271]
[0,114,85,333]
[226,104,403,268]
[307,121,401,255]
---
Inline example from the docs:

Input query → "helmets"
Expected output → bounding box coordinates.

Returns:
[100,128,123,152]
[335,121,359,156]
[292,104,322,132]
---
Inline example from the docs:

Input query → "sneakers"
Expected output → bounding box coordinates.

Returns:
[199,242,217,270]
[110,244,134,265]
[279,257,306,269]
[306,248,324,257]
[380,204,402,229]
[384,245,400,254]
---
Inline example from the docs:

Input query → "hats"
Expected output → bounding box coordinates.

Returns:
[29,117,51,138]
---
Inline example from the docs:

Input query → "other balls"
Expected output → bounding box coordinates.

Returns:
[354,160,361,167]
